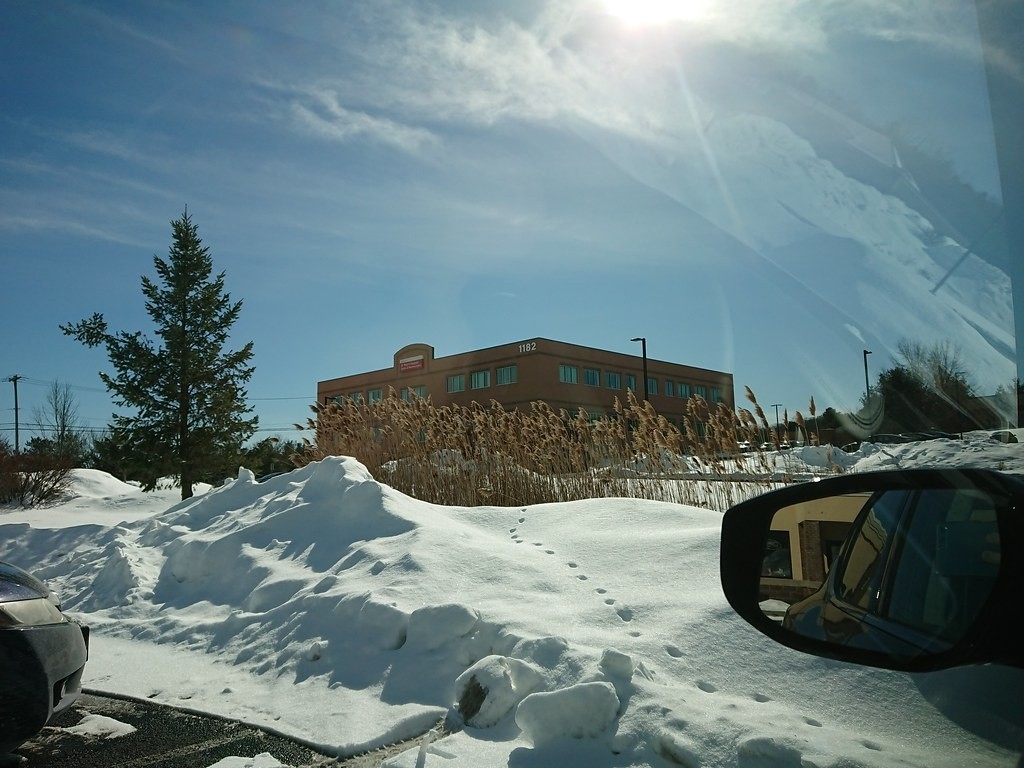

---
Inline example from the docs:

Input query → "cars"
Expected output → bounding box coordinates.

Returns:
[839,430,959,452]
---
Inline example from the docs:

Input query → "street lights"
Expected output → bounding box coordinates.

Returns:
[771,404,782,441]
[954,370,966,440]
[630,337,648,401]
[862,350,875,442]
